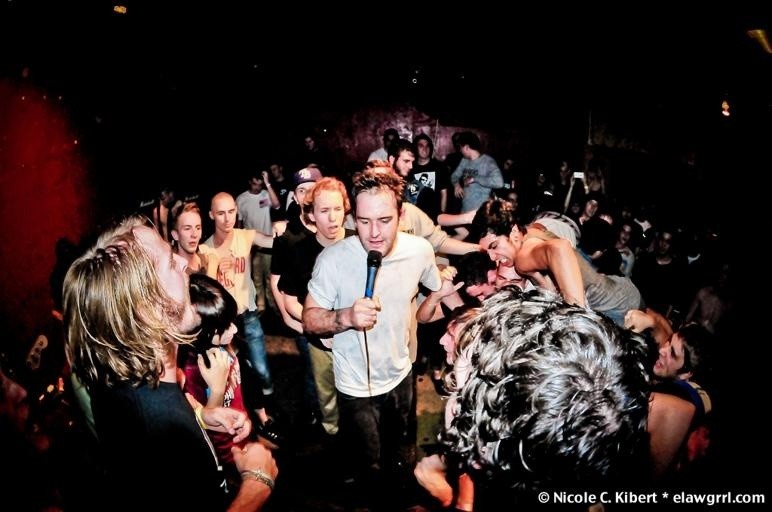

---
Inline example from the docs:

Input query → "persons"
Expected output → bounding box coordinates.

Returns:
[170,201,208,277]
[390,165,447,398]
[302,120,446,165]
[197,192,275,396]
[236,169,281,314]
[277,176,360,434]
[270,167,323,406]
[176,273,259,465]
[263,162,295,238]
[152,191,182,242]
[302,165,447,512]
[447,129,771,511]
[62,216,280,512]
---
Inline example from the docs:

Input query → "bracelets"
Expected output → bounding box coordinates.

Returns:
[265,183,271,187]
[194,408,208,430]
[237,470,276,492]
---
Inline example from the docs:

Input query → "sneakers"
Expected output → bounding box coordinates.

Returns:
[260,419,280,446]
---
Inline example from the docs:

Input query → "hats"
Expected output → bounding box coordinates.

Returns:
[293,163,322,190]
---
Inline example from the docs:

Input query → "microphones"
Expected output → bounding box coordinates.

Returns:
[364,250,382,298]
